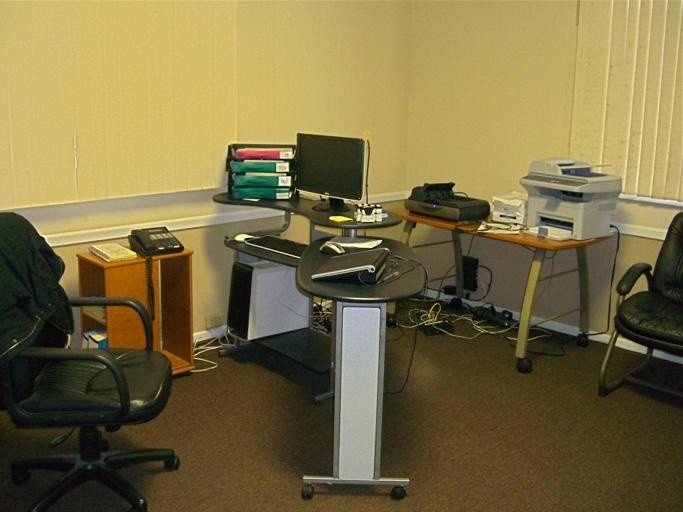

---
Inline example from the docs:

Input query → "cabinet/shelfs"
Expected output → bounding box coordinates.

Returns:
[77,245,196,381]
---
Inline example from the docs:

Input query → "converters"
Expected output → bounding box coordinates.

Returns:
[502,310,512,320]
[462,254,479,292]
[442,284,457,296]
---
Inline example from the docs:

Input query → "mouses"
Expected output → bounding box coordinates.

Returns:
[235,233,253,242]
[320,242,346,254]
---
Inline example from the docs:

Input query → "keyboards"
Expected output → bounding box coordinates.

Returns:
[246,234,308,259]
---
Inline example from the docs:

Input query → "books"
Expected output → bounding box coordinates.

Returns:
[80,294,107,321]
[87,242,138,262]
[229,184,297,201]
[80,325,105,349]
[87,332,107,351]
[232,172,297,187]
[229,159,291,174]
[234,148,296,160]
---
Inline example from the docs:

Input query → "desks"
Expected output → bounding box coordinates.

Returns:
[210,189,426,503]
[384,196,615,373]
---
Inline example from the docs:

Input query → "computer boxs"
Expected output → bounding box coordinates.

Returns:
[225,262,314,341]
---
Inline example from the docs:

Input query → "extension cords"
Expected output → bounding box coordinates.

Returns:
[475,304,510,327]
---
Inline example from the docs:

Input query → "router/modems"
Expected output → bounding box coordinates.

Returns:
[419,320,454,336]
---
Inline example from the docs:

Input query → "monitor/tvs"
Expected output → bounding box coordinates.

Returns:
[293,133,368,213]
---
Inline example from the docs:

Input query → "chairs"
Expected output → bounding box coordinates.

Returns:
[600,212,682,399]
[0,209,180,511]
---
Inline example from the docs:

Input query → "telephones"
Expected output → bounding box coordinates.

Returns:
[127,226,184,255]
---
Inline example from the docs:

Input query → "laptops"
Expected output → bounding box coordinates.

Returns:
[311,247,391,283]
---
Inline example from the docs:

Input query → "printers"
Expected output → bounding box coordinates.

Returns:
[405,182,490,221]
[519,157,623,242]
